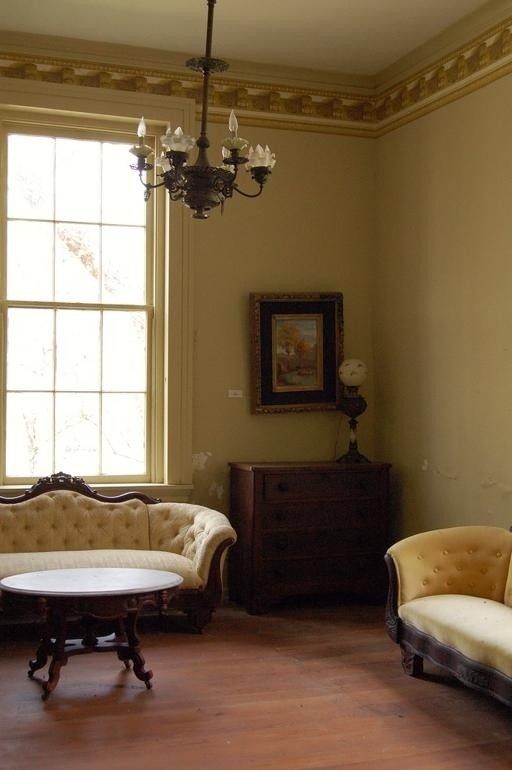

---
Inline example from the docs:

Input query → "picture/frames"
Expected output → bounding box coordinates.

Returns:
[246,287,346,418]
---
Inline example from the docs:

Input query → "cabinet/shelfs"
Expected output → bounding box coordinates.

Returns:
[224,458,394,617]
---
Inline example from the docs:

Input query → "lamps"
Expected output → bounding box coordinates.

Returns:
[336,357,371,464]
[127,1,278,220]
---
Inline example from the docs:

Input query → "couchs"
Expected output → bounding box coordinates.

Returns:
[1,472,238,637]
[378,524,512,712]
